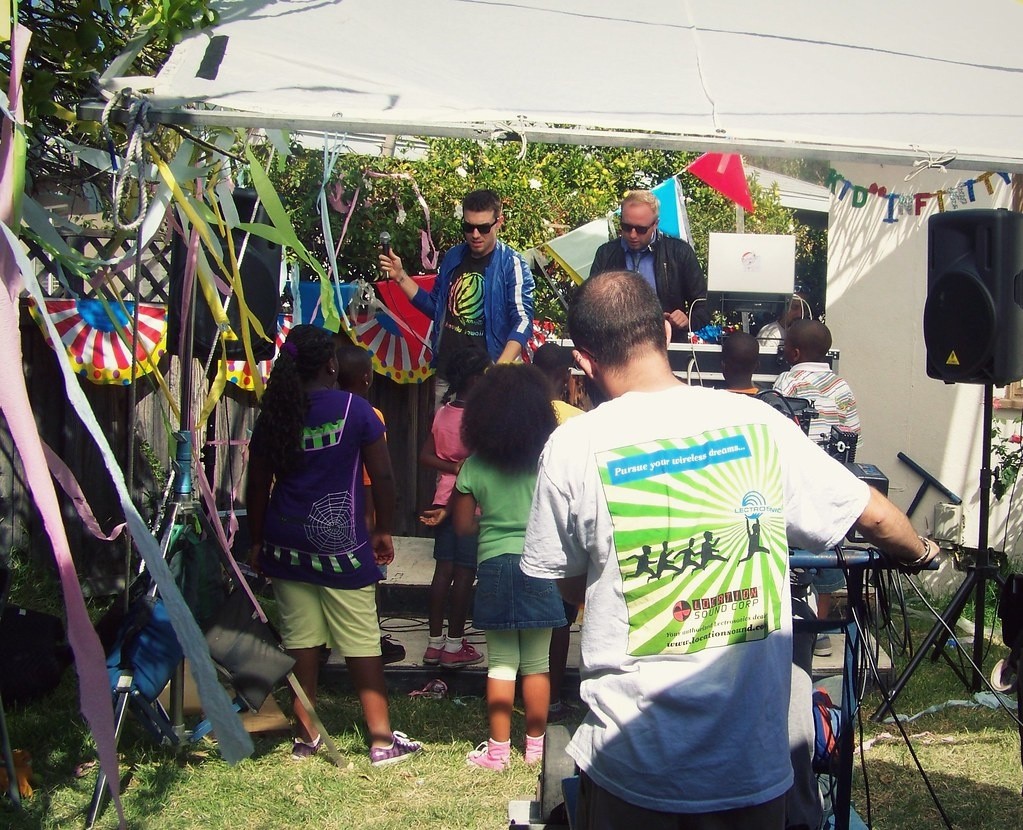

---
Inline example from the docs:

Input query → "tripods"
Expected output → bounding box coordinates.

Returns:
[86,274,254,830]
[870,382,1022,724]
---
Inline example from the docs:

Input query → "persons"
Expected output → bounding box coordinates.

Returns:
[690,290,864,466]
[329,346,408,665]
[574,191,712,345]
[415,343,589,775]
[246,326,428,767]
[517,270,941,830]
[378,191,534,403]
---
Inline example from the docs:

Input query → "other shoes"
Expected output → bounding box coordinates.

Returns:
[814,633,833,656]
[380,634,406,662]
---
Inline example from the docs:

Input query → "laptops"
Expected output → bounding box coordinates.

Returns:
[702,232,796,335]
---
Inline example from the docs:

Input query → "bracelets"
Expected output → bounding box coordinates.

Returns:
[895,534,933,570]
[397,270,408,286]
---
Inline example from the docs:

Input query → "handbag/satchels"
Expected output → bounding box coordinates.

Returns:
[203,589,297,717]
[105,592,185,704]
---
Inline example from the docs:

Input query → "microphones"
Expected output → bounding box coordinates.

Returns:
[379,232,391,283]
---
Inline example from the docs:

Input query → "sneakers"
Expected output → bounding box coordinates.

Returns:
[439,638,484,668]
[465,737,511,772]
[423,644,445,663]
[291,733,323,761]
[369,730,423,767]
[524,732,546,764]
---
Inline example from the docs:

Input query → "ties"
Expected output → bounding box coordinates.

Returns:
[629,249,649,274]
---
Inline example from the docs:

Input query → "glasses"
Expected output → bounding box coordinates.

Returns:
[460,215,497,234]
[794,284,812,294]
[620,217,657,234]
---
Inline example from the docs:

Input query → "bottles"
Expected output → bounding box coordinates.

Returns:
[944,639,957,648]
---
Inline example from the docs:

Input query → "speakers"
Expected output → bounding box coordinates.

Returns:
[930,206,1023,382]
[166,187,288,360]
